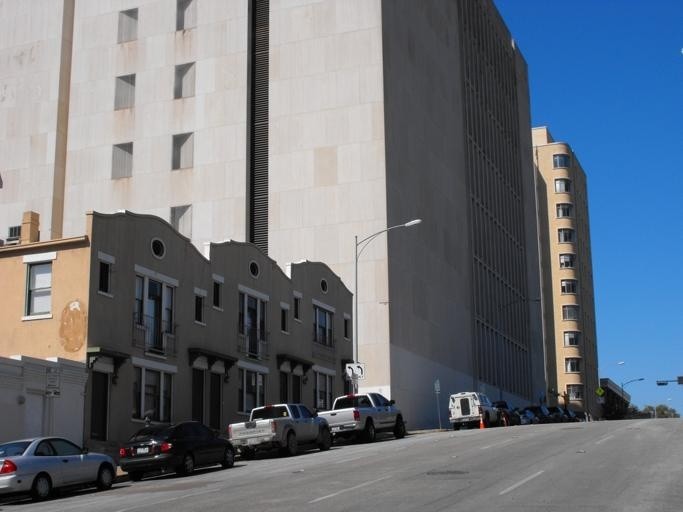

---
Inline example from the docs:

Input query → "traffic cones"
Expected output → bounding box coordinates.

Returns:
[480,414,486,429]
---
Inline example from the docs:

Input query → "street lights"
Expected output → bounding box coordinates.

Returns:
[598,361,624,421]
[352,219,422,406]
[622,379,644,419]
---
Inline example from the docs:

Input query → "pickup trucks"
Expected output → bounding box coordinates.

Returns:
[315,393,406,441]
[229,402,332,456]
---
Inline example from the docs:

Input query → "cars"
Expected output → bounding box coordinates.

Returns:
[491,400,585,426]
[0,436,117,501]
[120,419,235,480]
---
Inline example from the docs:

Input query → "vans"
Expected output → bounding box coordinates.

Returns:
[449,392,500,430]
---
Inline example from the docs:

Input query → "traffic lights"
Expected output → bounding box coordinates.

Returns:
[657,382,669,385]
